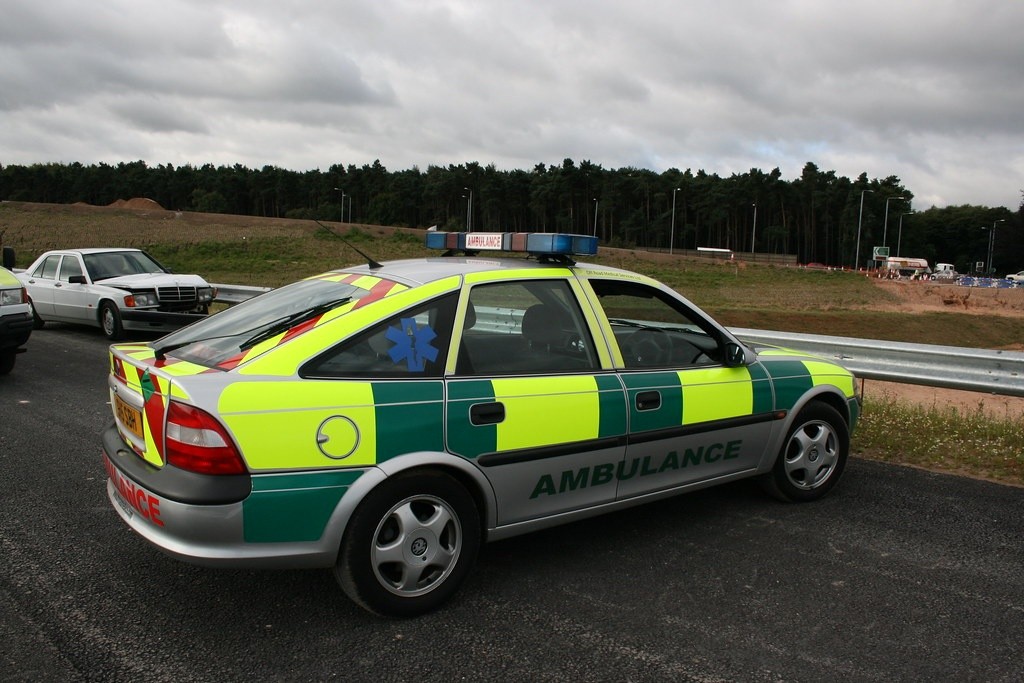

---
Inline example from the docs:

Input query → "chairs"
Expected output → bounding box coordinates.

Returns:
[424,300,475,375]
[512,304,562,373]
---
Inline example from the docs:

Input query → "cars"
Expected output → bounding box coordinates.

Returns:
[0,265,34,374]
[1003,271,1024,286]
[930,269,960,281]
[13,246,218,342]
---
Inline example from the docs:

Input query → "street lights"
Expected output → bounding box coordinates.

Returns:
[855,190,873,271]
[462,195,470,231]
[981,225,992,274]
[897,211,915,257]
[593,198,598,236]
[751,203,757,254]
[881,196,905,269]
[343,195,352,223]
[988,219,1006,276]
[464,187,472,232]
[335,187,344,222]
[670,188,681,255]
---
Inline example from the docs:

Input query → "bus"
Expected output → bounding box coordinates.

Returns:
[885,256,933,279]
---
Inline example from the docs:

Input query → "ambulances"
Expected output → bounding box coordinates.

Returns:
[96,229,863,624]
